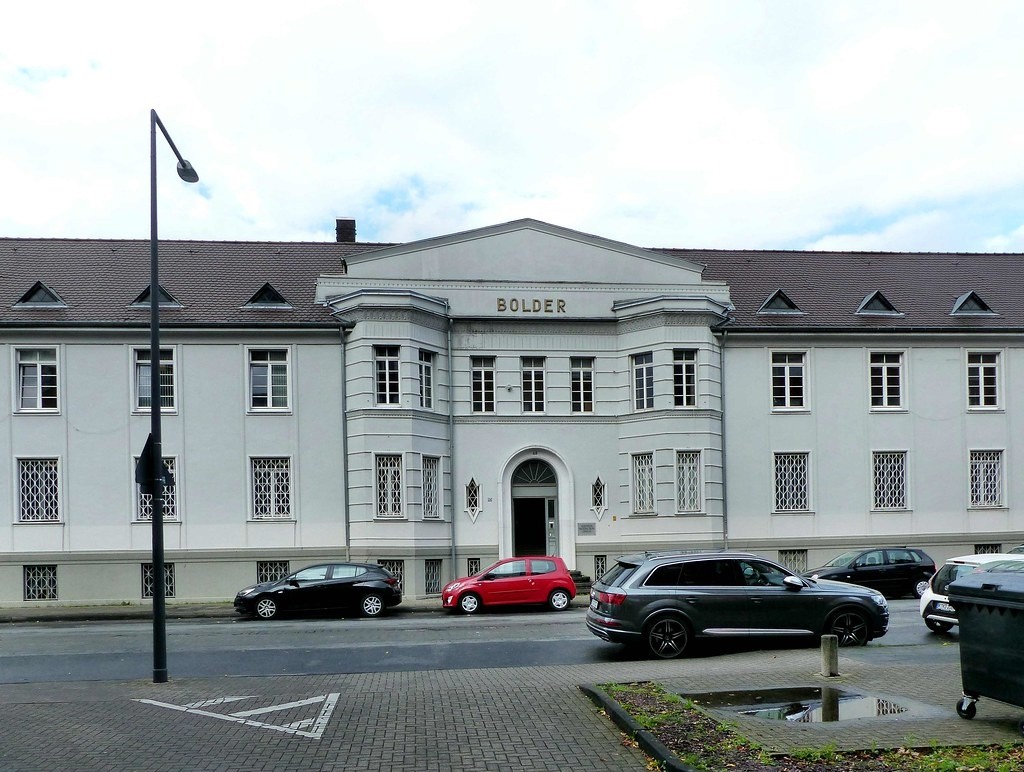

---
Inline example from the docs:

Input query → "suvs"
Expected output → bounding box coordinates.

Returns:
[797,545,937,599]
[586,551,891,659]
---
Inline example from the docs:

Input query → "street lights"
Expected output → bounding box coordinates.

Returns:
[142,107,199,683]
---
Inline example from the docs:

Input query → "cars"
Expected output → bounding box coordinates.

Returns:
[233,560,404,621]
[442,557,577,615]
[1006,543,1024,554]
[918,553,1023,635]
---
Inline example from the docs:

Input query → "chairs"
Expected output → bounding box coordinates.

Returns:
[867,557,876,564]
[894,553,906,563]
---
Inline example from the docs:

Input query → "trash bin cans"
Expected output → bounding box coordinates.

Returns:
[947,559,1024,720]
